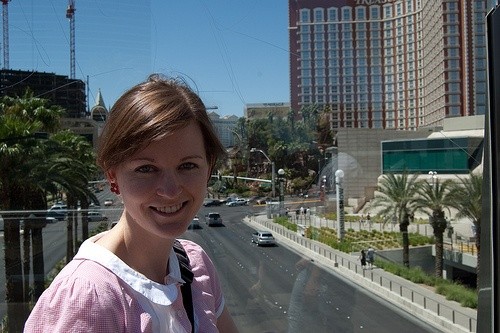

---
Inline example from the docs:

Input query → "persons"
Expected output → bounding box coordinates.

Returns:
[357,249,367,270]
[23,80,239,333]
[284,207,311,220]
[360,213,373,227]
[366,246,375,270]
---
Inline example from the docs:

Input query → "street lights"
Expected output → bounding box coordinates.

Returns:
[335,170,347,243]
[277,168,286,208]
[249,148,275,197]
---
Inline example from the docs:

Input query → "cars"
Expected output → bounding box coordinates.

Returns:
[247,196,259,204]
[87,213,108,222]
[46,204,67,216]
[252,231,275,246]
[257,197,272,205]
[206,213,222,226]
[226,198,247,207]
[192,215,200,225]
[204,200,220,208]
[104,199,113,206]
